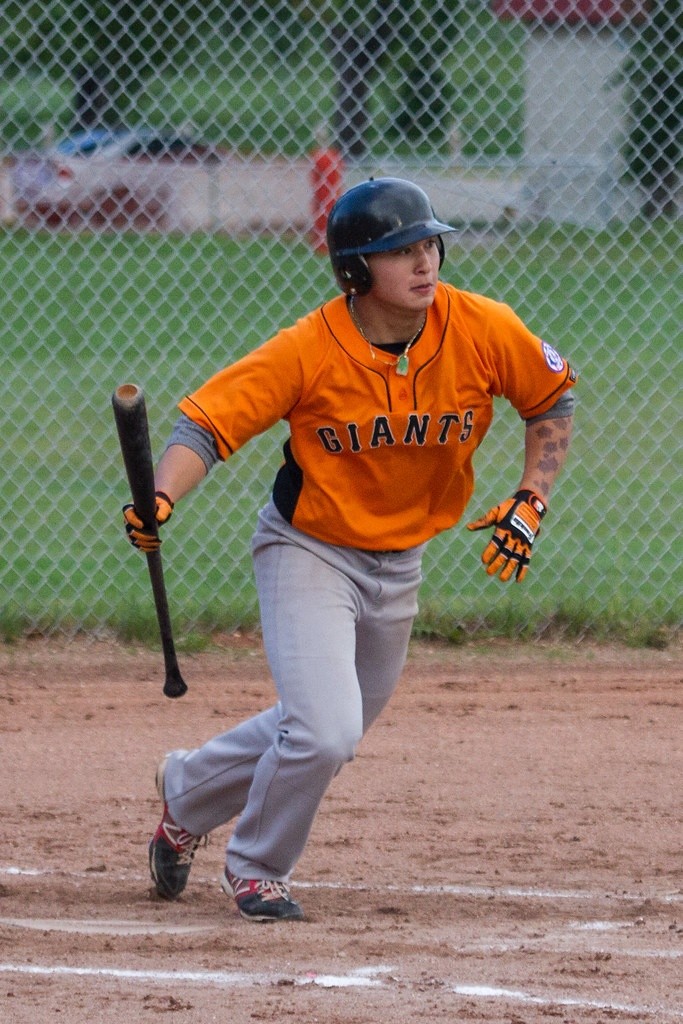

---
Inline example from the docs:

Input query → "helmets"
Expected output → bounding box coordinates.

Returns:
[326,177,459,297]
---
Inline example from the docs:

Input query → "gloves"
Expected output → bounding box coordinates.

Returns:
[467,489,547,582]
[121,492,173,552]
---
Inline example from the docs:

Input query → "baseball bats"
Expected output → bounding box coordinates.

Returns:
[112,381,190,697]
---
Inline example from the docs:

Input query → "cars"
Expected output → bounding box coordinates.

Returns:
[5,128,218,233]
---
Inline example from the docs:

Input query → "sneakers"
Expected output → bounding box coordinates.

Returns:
[220,867,304,924]
[149,755,210,901]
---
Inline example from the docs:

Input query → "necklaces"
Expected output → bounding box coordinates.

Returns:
[349,294,426,377]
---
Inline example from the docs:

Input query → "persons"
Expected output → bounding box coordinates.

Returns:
[309,126,345,256]
[122,177,580,923]
